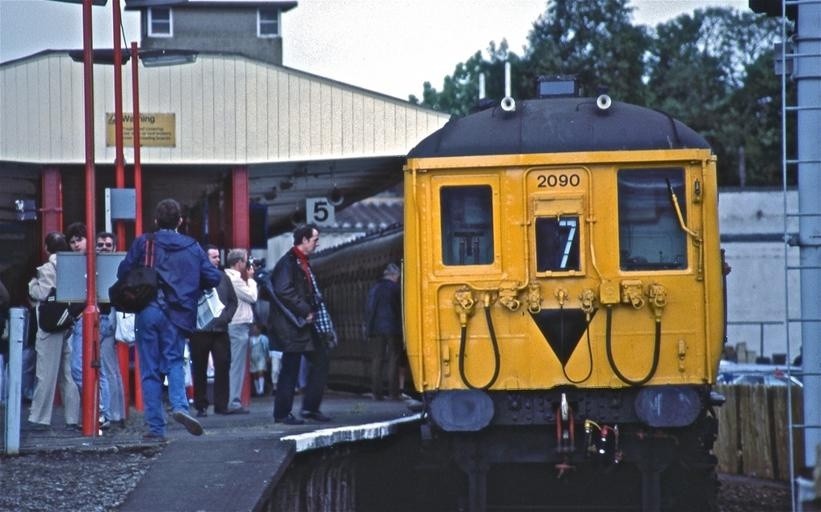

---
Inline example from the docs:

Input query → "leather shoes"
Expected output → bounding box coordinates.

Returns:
[300,411,331,422]
[274,413,304,425]
[198,405,248,417]
[26,416,111,433]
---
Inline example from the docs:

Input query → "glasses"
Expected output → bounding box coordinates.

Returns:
[96,241,115,248]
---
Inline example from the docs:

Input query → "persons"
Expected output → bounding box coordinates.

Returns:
[269,222,337,426]
[1,221,308,431]
[117,198,224,440]
[375,261,410,399]
[363,261,413,402]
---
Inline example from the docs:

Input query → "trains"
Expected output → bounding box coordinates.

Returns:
[303,58,734,512]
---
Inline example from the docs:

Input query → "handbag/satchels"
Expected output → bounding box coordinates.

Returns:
[313,302,333,343]
[197,286,226,332]
[39,287,72,334]
[109,265,157,314]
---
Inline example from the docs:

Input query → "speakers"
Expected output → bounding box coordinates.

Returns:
[251,202,268,250]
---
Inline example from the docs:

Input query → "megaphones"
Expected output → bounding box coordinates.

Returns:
[327,187,344,206]
[290,207,305,226]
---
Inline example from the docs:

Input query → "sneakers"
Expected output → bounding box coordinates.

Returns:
[143,432,166,443]
[174,412,202,436]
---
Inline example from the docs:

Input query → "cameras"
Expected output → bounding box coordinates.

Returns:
[248,255,266,269]
